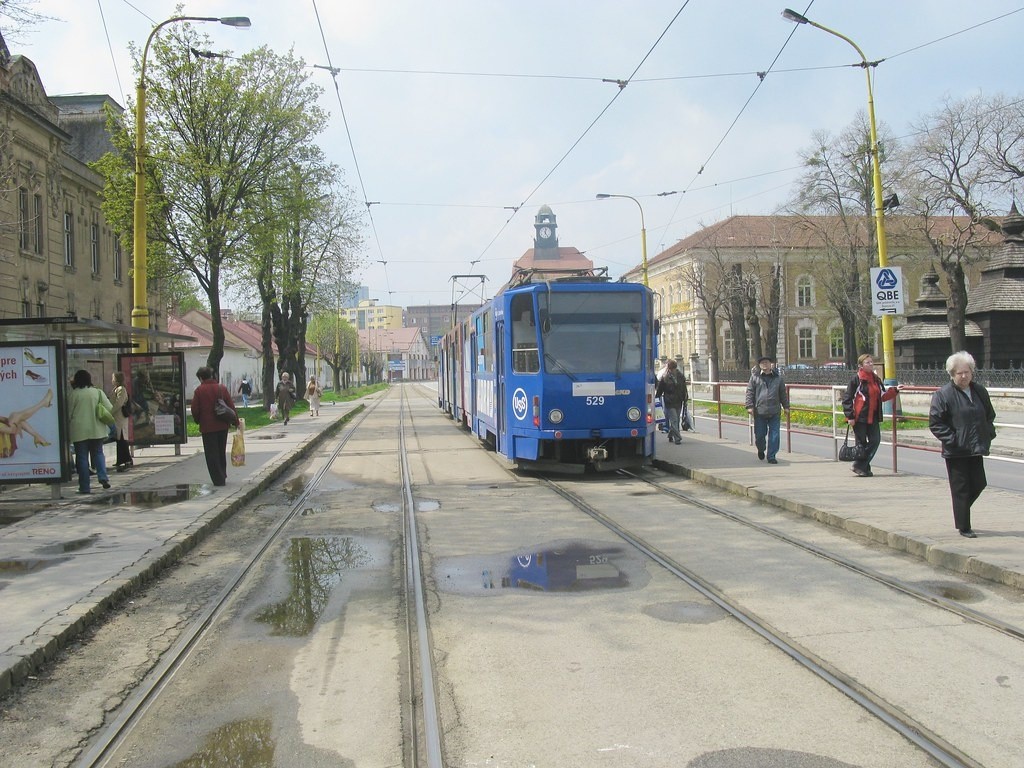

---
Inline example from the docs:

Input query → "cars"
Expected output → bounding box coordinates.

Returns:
[751,362,847,373]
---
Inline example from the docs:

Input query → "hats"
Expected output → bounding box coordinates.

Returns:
[758,357,771,363]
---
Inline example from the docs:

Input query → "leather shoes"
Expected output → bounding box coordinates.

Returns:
[959,529,977,537]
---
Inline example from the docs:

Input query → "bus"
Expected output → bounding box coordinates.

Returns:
[434,272,662,474]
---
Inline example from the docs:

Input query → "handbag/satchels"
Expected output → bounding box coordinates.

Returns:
[96,402,116,426]
[214,399,238,425]
[231,430,246,467]
[682,404,692,431]
[269,403,280,420]
[839,425,867,461]
[122,399,141,418]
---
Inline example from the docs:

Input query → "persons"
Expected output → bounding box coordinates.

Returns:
[238,378,251,408]
[928,350,997,539]
[0,389,54,458]
[745,356,790,464]
[109,372,133,467]
[191,366,240,486]
[842,353,904,477]
[67,370,113,495]
[274,371,296,426]
[151,390,182,431]
[130,369,157,426]
[303,375,323,417]
[654,354,689,445]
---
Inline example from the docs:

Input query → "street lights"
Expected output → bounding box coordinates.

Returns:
[595,193,649,287]
[127,16,251,353]
[782,8,902,418]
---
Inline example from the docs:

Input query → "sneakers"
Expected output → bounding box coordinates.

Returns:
[100,480,111,488]
[757,450,765,460]
[669,437,673,442]
[767,458,777,464]
[850,463,872,476]
[675,440,681,445]
[115,459,134,468]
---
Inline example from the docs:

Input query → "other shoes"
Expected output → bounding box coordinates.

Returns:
[284,422,287,425]
